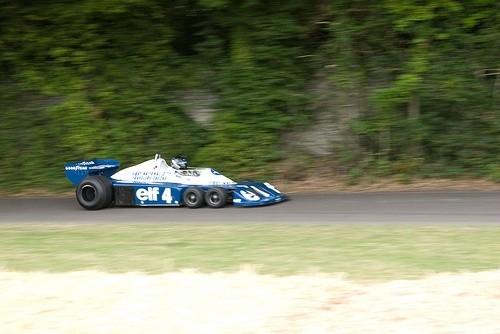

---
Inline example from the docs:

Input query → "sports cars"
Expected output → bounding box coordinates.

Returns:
[64,154,288,209]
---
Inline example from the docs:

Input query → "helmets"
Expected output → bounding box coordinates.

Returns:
[172,156,188,169]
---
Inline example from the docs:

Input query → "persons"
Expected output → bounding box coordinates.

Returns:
[171,155,188,171]
